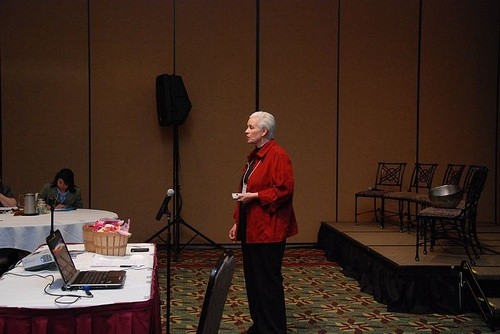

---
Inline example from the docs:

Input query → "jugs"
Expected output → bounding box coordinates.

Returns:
[18,192,40,214]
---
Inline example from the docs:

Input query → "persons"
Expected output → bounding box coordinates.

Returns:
[0,184,17,207]
[36,168,84,208]
[229,112,298,334]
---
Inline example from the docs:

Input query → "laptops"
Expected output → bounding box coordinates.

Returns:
[45,229,126,289]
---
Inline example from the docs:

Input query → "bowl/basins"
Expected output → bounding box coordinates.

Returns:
[427,184,463,209]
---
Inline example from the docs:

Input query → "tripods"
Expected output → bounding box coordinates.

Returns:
[144,126,224,253]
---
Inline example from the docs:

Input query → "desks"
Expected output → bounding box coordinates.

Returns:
[0,207,161,334]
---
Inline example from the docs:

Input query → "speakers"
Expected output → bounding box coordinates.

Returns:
[156,74,191,127]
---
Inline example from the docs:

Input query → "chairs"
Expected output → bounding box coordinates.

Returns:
[196,251,235,334]
[355,161,489,267]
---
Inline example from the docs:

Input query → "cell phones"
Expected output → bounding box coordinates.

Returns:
[232,193,241,199]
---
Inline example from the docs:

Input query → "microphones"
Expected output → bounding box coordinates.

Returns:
[155,189,174,221]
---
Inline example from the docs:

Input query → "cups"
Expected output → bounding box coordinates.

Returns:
[37,199,49,213]
